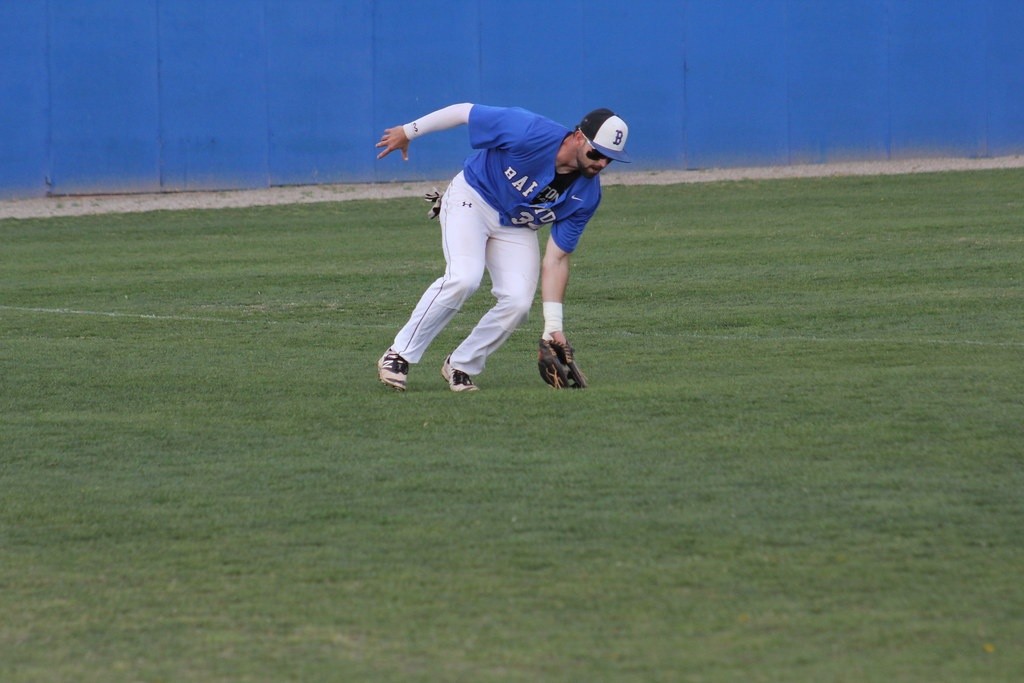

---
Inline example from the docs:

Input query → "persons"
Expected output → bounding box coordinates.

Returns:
[376,102,631,393]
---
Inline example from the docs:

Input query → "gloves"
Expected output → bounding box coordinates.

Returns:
[424,192,441,221]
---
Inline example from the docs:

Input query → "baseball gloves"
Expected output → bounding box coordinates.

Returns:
[537,337,589,388]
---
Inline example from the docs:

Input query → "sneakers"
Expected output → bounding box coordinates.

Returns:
[377,348,409,392]
[440,354,480,392]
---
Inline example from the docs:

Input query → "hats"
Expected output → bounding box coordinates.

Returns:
[579,108,633,163]
